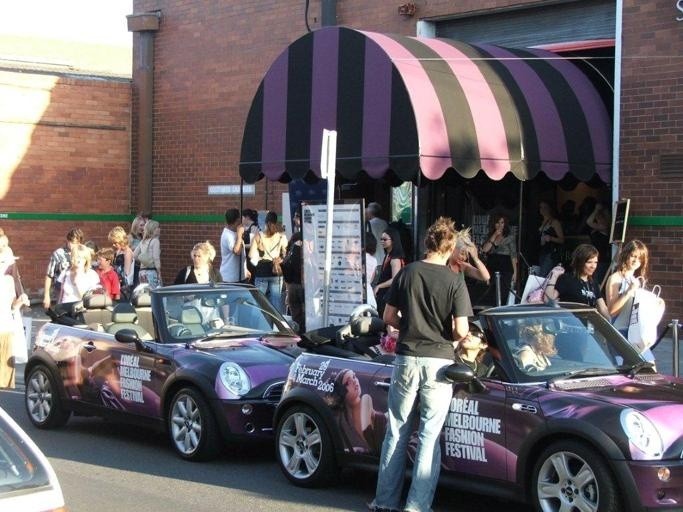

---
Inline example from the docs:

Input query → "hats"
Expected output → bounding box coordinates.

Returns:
[470,320,485,334]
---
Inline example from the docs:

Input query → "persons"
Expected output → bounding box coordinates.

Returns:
[0,228,31,390]
[325,369,517,483]
[175,240,217,284]
[219,208,305,335]
[367,217,475,512]
[366,200,665,371]
[75,336,160,417]
[42,216,162,308]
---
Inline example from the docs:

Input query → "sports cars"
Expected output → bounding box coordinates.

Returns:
[24,282,304,455]
[277,300,683,510]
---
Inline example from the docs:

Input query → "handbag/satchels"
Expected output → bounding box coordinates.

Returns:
[525,270,552,304]
[255,258,283,278]
[11,303,28,364]
[138,238,158,268]
[627,276,665,354]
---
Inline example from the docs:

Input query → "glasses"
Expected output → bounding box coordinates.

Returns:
[380,238,391,241]
[471,329,485,340]
